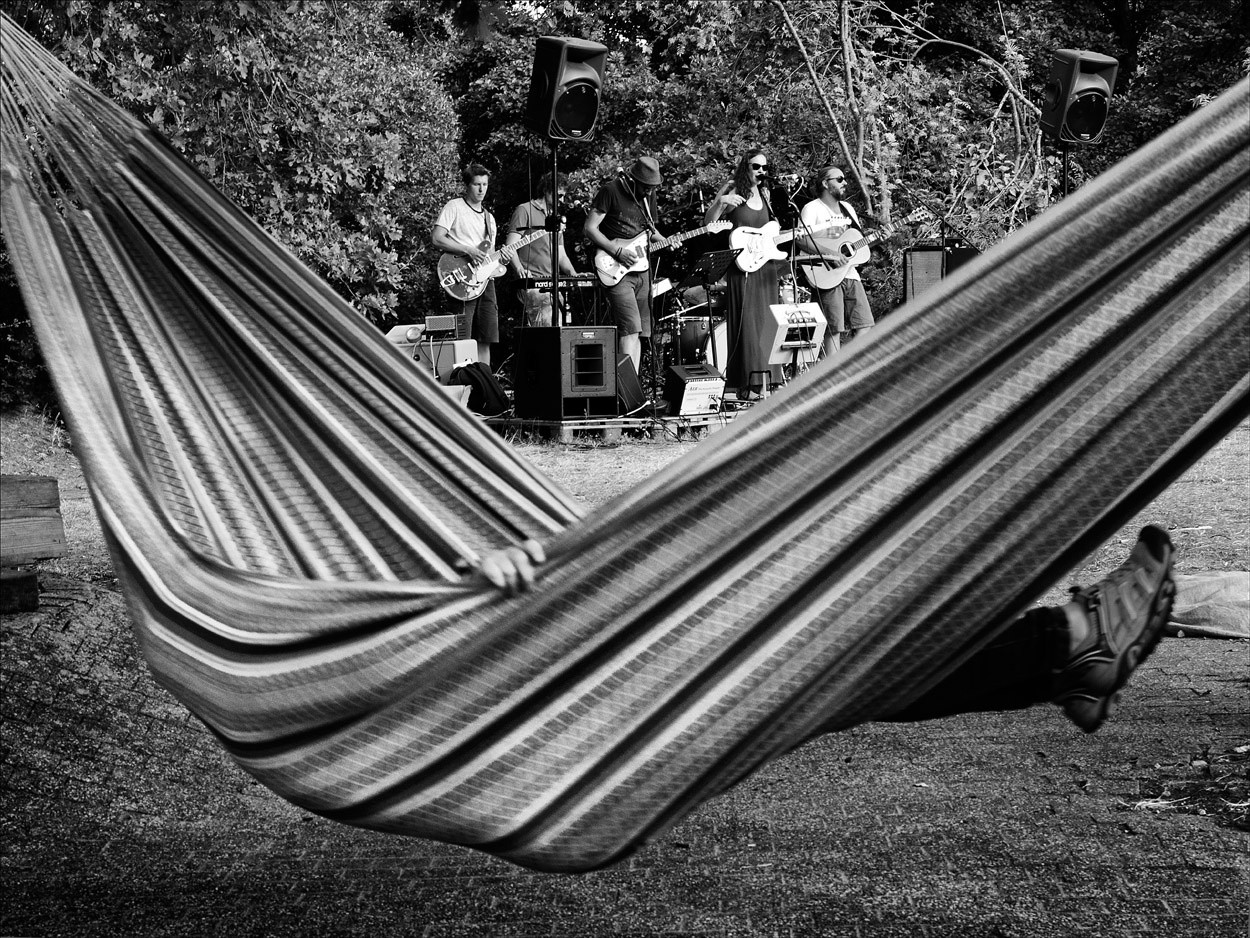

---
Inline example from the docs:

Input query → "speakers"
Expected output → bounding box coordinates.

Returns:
[903,246,979,304]
[523,36,608,141]
[1040,48,1119,144]
[662,363,727,416]
[513,325,618,420]
[615,352,647,416]
[395,339,479,386]
[759,301,828,363]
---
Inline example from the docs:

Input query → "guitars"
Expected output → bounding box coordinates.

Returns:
[437,222,569,300]
[800,205,932,288]
[595,219,734,286]
[726,214,852,275]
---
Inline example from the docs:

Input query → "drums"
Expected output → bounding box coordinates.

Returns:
[677,315,727,365]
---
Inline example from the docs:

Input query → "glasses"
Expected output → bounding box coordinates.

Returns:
[826,176,847,183]
[636,181,661,192]
[748,162,769,172]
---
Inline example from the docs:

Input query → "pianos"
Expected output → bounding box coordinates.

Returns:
[514,272,609,326]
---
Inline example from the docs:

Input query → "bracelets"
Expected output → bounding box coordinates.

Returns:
[614,247,622,259]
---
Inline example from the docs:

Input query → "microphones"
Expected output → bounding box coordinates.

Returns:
[780,176,804,182]
[758,173,780,181]
[895,178,912,197]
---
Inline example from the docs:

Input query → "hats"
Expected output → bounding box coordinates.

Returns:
[626,156,664,186]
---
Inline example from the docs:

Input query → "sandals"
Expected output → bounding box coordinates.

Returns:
[736,388,763,401]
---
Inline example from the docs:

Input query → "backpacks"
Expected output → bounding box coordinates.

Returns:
[448,361,511,416]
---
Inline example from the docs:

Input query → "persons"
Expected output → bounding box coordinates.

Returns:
[463,525,1177,733]
[705,149,785,400]
[432,164,514,366]
[585,157,683,376]
[507,172,594,326]
[798,166,891,357]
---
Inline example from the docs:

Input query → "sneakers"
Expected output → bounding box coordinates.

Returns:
[1048,523,1179,734]
